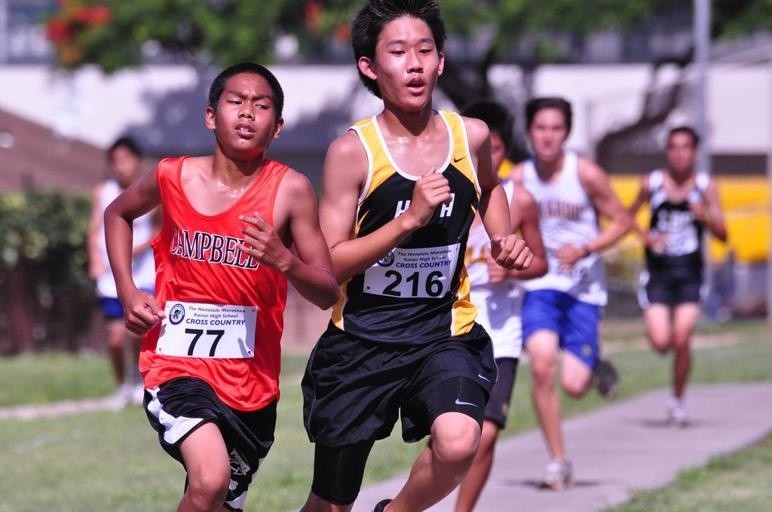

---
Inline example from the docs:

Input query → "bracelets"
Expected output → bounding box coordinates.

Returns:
[580,243,593,257]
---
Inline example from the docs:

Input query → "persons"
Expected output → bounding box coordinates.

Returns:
[441,99,556,512]
[510,94,640,493]
[630,124,730,425]
[298,0,537,512]
[85,131,160,410]
[101,58,345,512]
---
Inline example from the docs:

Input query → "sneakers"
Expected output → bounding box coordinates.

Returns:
[537,458,572,491]
[592,356,619,402]
